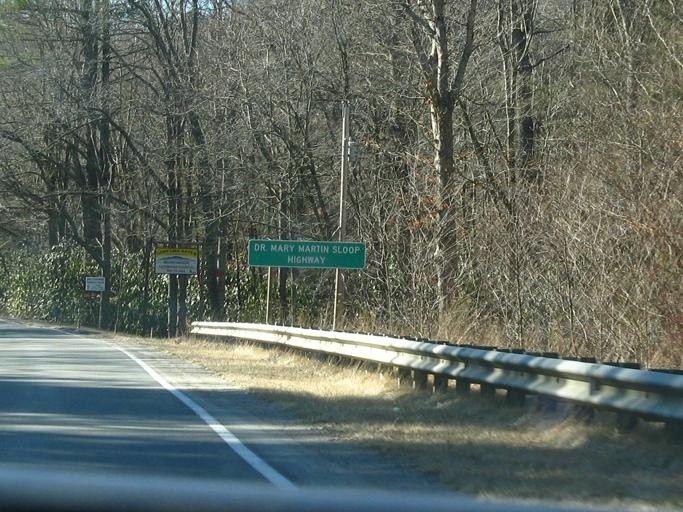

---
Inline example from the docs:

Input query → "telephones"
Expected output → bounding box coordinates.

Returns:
[248,239,366,269]
